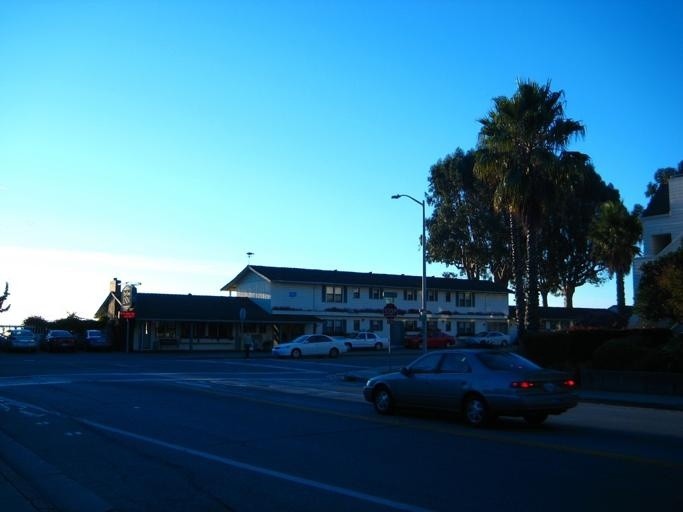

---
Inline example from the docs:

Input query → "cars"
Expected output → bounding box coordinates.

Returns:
[403,328,457,350]
[336,331,392,351]
[0,328,42,351]
[77,329,114,351]
[458,331,513,347]
[362,346,579,430]
[40,329,78,352]
[272,332,349,358]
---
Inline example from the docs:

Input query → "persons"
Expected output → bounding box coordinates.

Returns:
[242,331,252,358]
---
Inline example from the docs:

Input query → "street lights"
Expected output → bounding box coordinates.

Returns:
[389,191,430,359]
